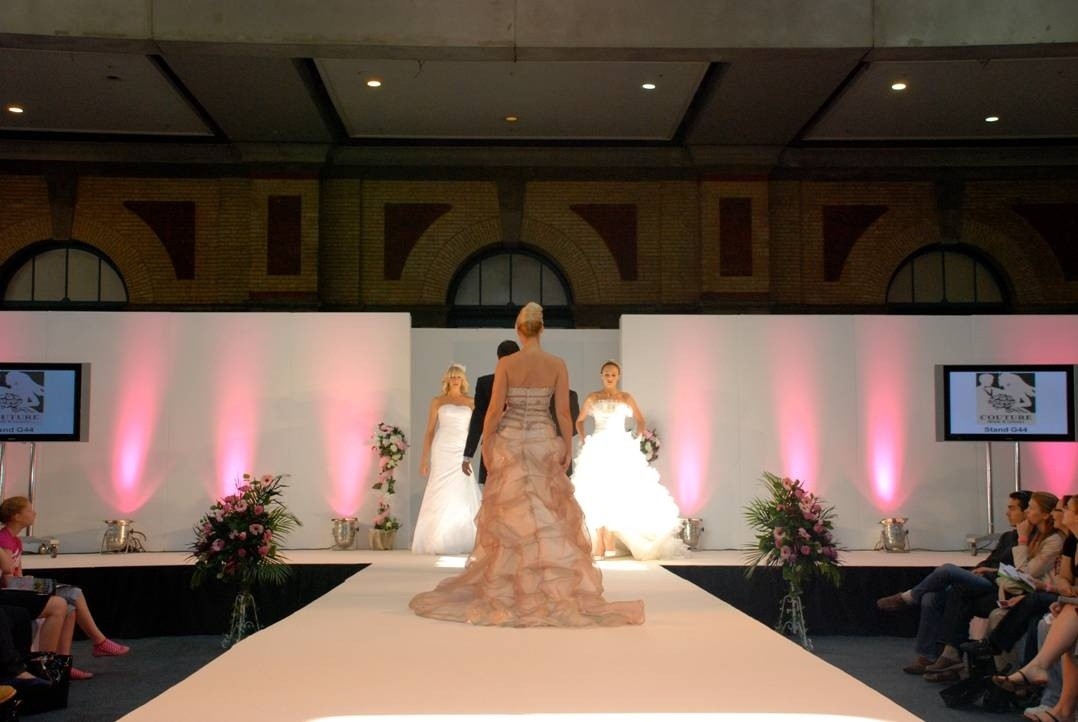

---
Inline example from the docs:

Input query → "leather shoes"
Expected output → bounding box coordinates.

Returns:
[923,672,952,683]
[926,656,963,671]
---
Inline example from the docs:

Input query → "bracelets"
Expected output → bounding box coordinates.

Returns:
[463,457,471,462]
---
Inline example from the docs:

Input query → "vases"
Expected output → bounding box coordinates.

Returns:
[367,530,396,550]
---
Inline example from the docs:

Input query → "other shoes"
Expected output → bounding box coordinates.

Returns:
[7,677,52,689]
[71,667,93,679]
[92,638,130,657]
[876,591,918,611]
[1024,704,1060,722]
[902,656,935,674]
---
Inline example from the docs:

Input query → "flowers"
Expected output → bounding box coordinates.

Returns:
[183,473,304,652]
[738,469,849,596]
[640,430,661,463]
[371,424,411,531]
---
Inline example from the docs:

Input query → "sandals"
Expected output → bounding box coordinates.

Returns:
[992,671,1049,691]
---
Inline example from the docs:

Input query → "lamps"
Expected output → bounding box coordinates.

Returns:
[331,518,359,551]
[874,517,912,553]
[680,518,705,551]
[100,520,139,555]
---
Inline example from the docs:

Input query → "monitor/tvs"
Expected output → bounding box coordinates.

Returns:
[0,362,83,442]
[941,363,1078,441]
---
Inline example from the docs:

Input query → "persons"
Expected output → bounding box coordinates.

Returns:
[479,303,573,596]
[0,497,131,680]
[570,359,645,559]
[996,373,1037,423]
[875,490,1078,722]
[462,339,520,487]
[0,544,69,722]
[411,362,482,557]
[976,374,1004,421]
[548,386,581,486]
[1,370,46,420]
